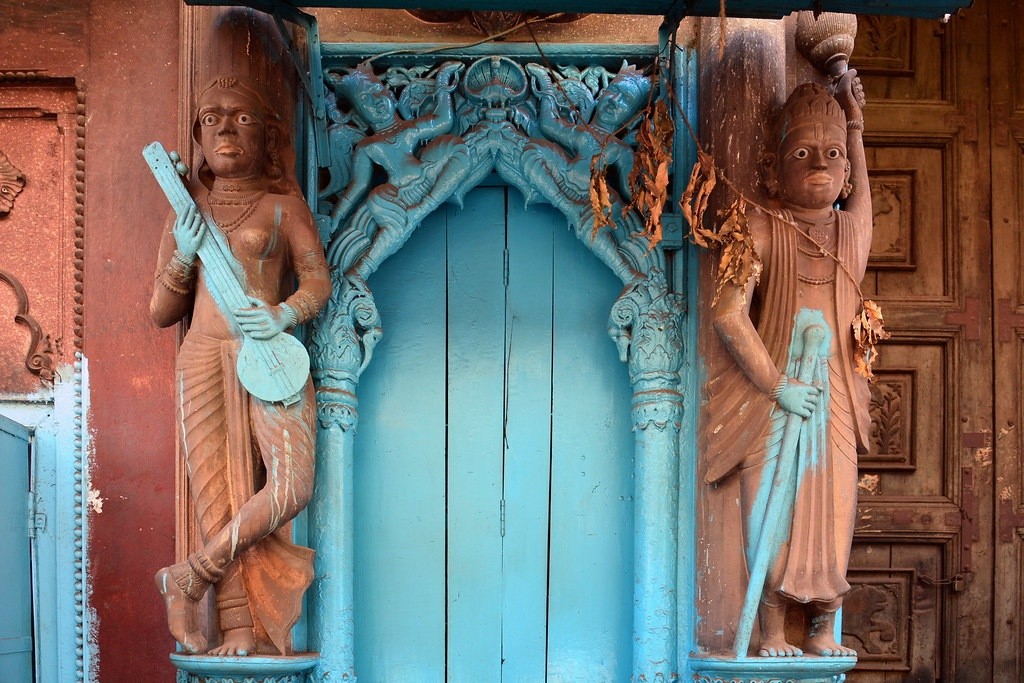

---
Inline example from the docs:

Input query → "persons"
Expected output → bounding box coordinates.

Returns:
[711,66,875,660]
[149,11,331,658]
[523,62,657,211]
[319,56,463,243]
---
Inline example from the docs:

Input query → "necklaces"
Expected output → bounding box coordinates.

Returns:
[205,190,265,204]
[790,209,836,244]
[214,201,257,234]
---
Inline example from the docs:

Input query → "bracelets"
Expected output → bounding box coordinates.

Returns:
[279,300,298,328]
[847,119,864,134]
[156,275,189,295]
[288,294,311,323]
[300,293,320,318]
[164,252,194,284]
[769,375,788,401]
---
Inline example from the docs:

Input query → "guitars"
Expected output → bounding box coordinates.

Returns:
[141,140,312,409]
